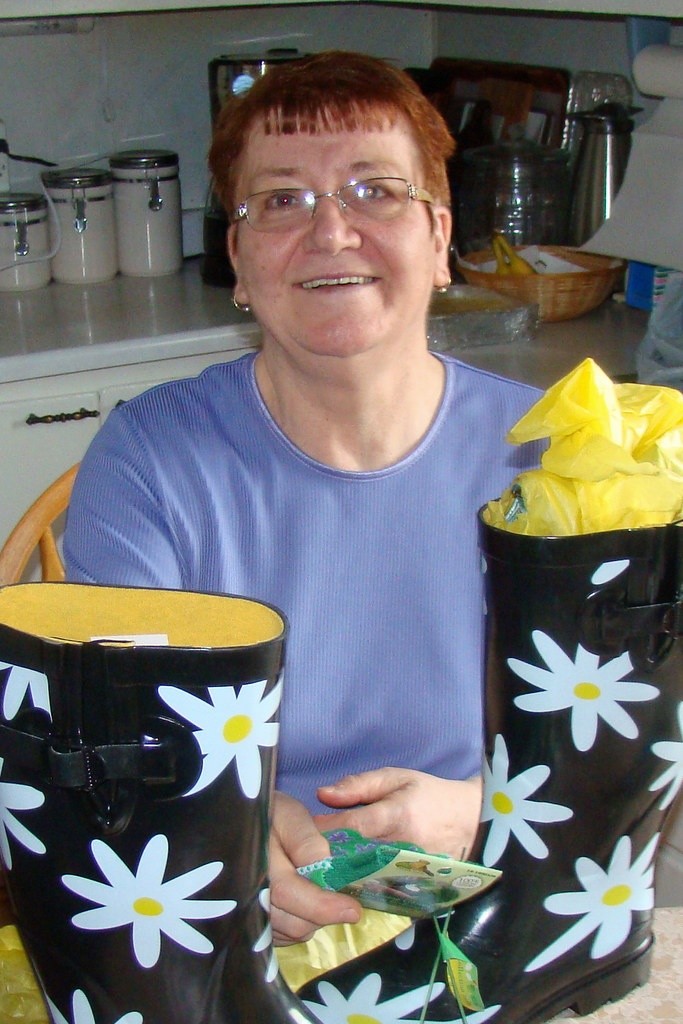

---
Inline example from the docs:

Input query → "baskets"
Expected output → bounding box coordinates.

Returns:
[454,243,626,323]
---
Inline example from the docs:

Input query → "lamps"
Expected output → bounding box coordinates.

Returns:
[0,16,95,37]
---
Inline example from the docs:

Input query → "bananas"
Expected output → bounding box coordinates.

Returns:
[492,235,538,274]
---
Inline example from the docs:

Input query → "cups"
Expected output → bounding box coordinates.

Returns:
[473,146,580,245]
[109,148,183,278]
[0,192,53,291]
[40,167,117,284]
[558,70,633,175]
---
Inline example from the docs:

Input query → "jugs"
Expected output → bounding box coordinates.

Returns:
[560,101,645,248]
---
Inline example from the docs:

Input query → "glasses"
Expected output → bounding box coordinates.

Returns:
[230,175,437,233]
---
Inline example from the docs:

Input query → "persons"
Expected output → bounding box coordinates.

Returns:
[63,52,548,948]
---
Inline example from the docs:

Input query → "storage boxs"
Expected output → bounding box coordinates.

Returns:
[423,281,540,350]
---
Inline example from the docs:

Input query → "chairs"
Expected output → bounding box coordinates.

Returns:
[0,460,80,589]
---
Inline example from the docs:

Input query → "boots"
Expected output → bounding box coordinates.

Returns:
[295,497,683,1024]
[0,583,334,1024]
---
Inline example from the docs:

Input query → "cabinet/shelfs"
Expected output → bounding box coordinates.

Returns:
[0,346,260,586]
